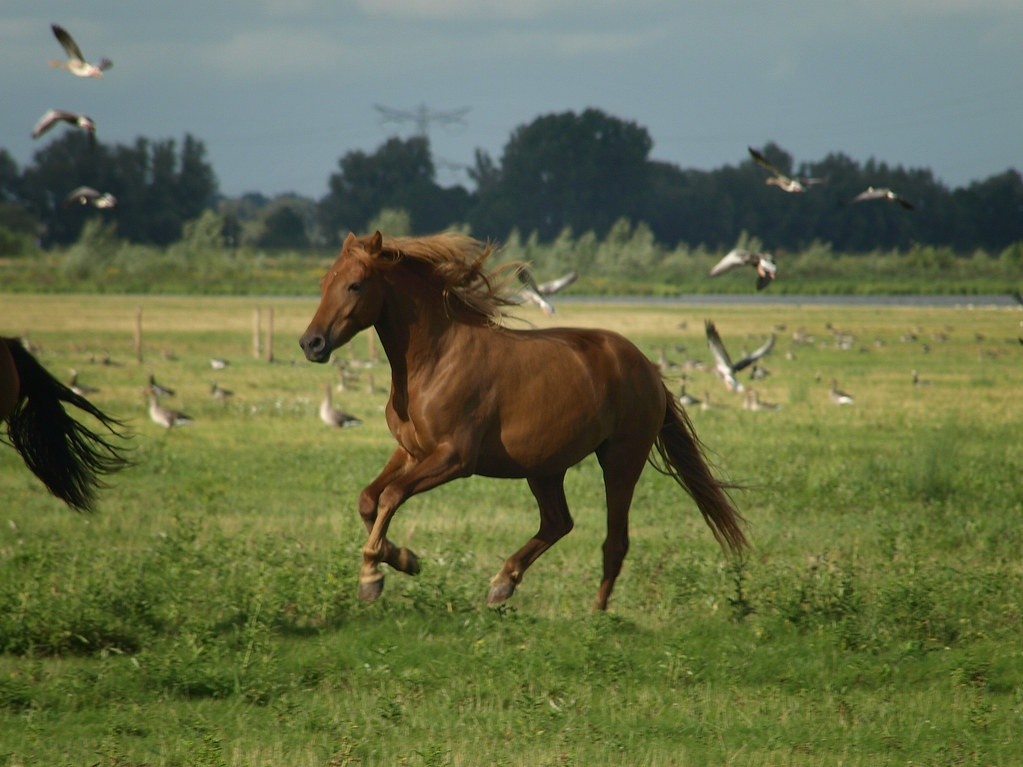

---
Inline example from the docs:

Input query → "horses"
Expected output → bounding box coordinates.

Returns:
[0,335,143,515]
[299,231,756,612]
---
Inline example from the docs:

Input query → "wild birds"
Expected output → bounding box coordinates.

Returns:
[33,110,95,153]
[67,353,384,428]
[68,186,116,210]
[855,186,913,210]
[46,23,114,80]
[747,146,825,191]
[512,246,950,410]
